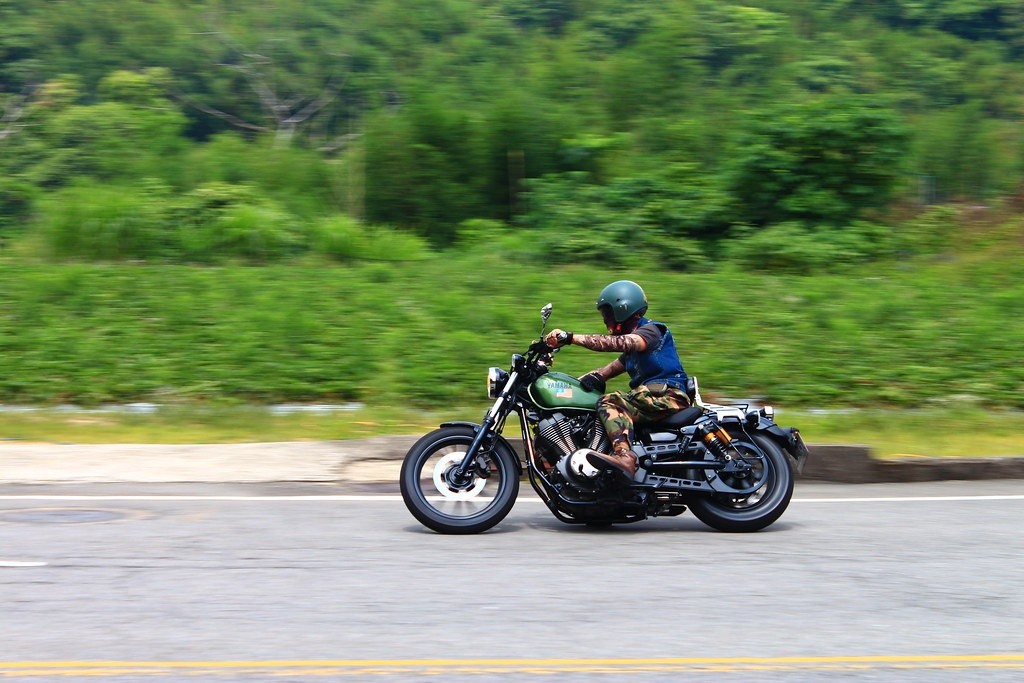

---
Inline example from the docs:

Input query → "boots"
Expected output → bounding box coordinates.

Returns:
[586,446,637,482]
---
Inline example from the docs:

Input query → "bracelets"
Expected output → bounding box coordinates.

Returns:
[565,331,573,345]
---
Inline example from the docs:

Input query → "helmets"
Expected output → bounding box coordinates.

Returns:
[595,281,649,321]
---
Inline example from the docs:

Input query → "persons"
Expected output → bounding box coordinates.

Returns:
[545,280,691,478]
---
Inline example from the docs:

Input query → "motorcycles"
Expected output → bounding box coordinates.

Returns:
[398,302,809,536]
